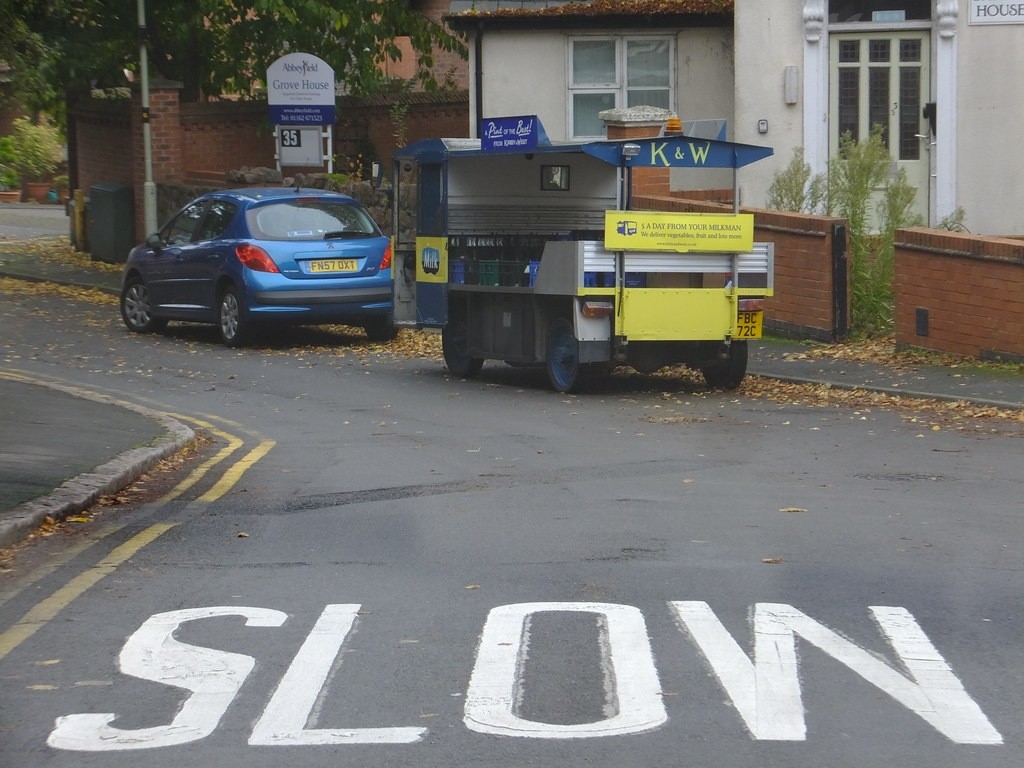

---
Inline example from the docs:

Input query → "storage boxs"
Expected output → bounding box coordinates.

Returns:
[449,257,649,288]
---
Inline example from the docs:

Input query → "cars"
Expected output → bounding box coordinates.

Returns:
[121,187,400,346]
